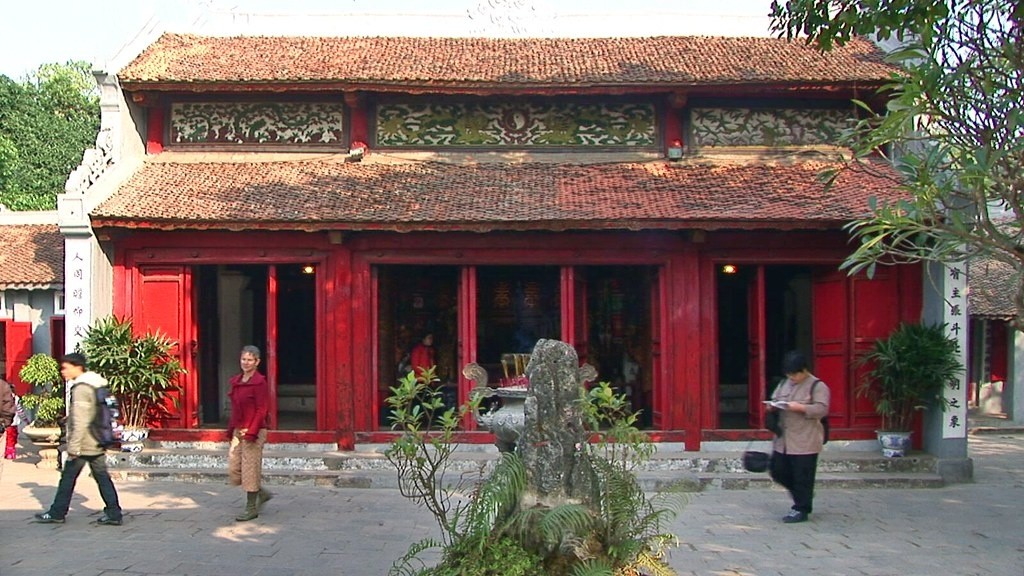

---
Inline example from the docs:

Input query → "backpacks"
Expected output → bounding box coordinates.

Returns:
[780,378,828,445]
[70,382,113,451]
[398,352,412,378]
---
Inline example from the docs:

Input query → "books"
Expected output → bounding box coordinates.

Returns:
[762,400,799,410]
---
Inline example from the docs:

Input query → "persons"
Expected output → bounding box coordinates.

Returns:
[766,351,830,522]
[35,353,123,525]
[226,345,273,521]
[0,379,29,460]
[410,331,436,421]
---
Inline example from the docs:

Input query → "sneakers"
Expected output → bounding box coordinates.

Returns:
[791,505,811,513]
[782,509,808,523]
[97,514,123,525]
[35,513,65,523]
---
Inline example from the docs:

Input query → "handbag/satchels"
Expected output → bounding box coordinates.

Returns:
[743,451,769,473]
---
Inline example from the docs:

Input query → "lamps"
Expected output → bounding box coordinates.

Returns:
[668,147,682,159]
[349,147,366,159]
[301,263,317,275]
[723,264,736,274]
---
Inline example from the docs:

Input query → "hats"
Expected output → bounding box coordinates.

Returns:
[764,410,783,438]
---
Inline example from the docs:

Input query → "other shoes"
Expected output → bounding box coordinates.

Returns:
[5,453,15,459]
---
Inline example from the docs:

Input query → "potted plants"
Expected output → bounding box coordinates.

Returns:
[850,320,966,460]
[19,353,67,470]
[80,314,190,454]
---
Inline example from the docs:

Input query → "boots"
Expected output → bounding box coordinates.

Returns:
[257,489,271,507]
[236,492,258,521]
